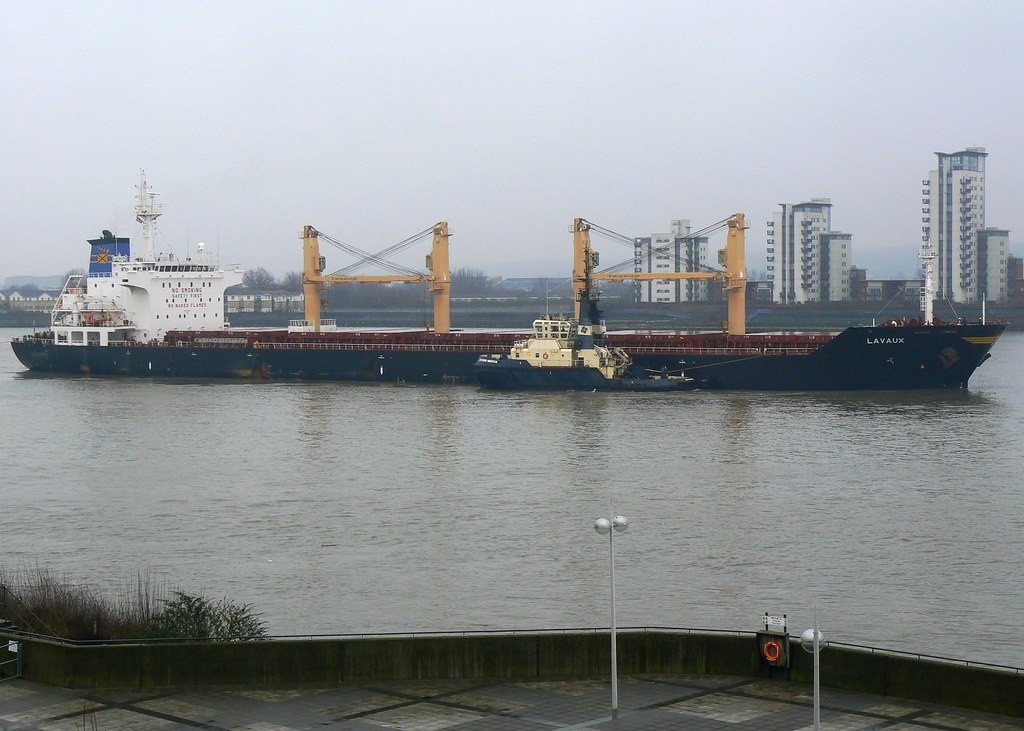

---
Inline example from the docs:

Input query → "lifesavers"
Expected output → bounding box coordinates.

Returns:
[764,642,780,661]
[543,354,548,359]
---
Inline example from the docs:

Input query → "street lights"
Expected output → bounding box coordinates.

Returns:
[802,605,828,730]
[595,496,629,718]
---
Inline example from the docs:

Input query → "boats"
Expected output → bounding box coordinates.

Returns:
[9,168,1008,392]
[472,243,696,394]
